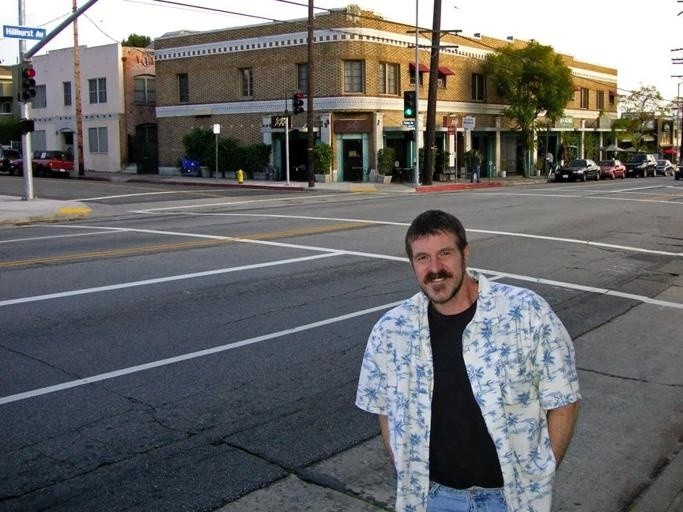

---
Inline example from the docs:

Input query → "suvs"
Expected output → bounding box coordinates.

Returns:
[622,154,658,178]
[0,149,21,174]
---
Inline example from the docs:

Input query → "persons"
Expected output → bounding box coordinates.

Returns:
[354,209,584,512]
[545,151,556,177]
[470,150,482,183]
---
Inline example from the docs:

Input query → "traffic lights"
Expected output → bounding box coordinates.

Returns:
[404,91,416,118]
[23,69,37,99]
[293,93,304,114]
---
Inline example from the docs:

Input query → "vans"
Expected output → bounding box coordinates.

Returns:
[9,150,73,177]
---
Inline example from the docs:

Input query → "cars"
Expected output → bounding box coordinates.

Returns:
[597,160,626,180]
[656,160,674,176]
[555,159,602,182]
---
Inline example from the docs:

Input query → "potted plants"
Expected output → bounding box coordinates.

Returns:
[182,127,395,184]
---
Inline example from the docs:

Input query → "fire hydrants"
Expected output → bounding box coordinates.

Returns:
[234,167,247,184]
